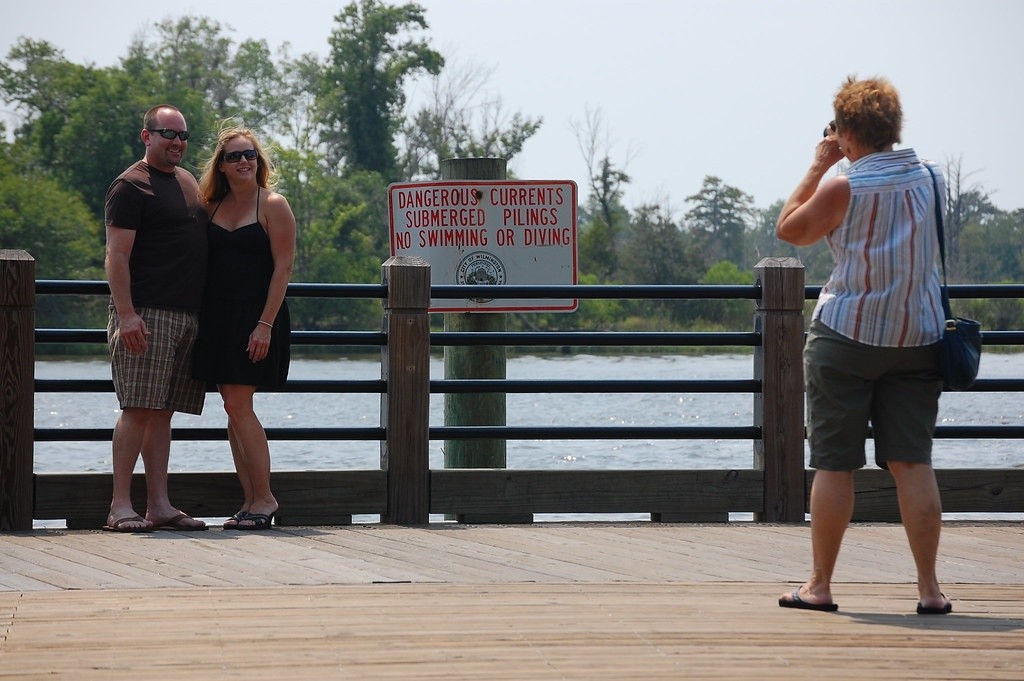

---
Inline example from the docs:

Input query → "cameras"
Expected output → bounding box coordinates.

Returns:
[824,120,836,137]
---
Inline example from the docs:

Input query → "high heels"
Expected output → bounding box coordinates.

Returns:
[223,506,280,529]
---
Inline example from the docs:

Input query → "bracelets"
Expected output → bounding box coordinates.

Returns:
[258,321,273,328]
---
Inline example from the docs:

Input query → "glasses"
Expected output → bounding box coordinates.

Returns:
[146,127,189,141]
[223,148,258,163]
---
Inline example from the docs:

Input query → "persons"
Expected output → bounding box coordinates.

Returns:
[775,74,954,615]
[202,128,297,529]
[102,103,209,534]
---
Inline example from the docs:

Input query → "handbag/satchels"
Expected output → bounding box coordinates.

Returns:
[933,317,983,392]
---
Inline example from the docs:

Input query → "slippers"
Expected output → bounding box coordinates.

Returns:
[102,515,155,532]
[153,514,210,531]
[917,592,952,614]
[779,586,838,611]
